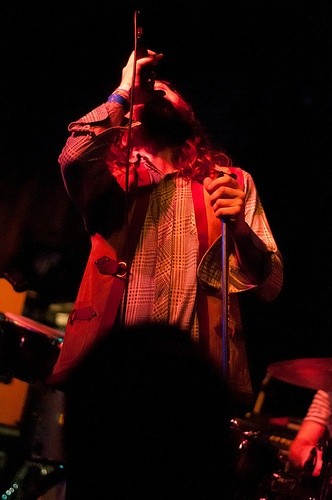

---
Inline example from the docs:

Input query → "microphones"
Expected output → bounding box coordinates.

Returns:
[134,11,158,96]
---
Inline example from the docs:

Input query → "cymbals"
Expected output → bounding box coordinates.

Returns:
[267,358,332,392]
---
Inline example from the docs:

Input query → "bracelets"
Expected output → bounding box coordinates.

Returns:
[107,92,131,115]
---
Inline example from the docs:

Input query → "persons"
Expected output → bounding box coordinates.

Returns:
[57,317,314,500]
[45,47,287,465]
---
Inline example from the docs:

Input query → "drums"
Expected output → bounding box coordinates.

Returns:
[1,308,67,391]
